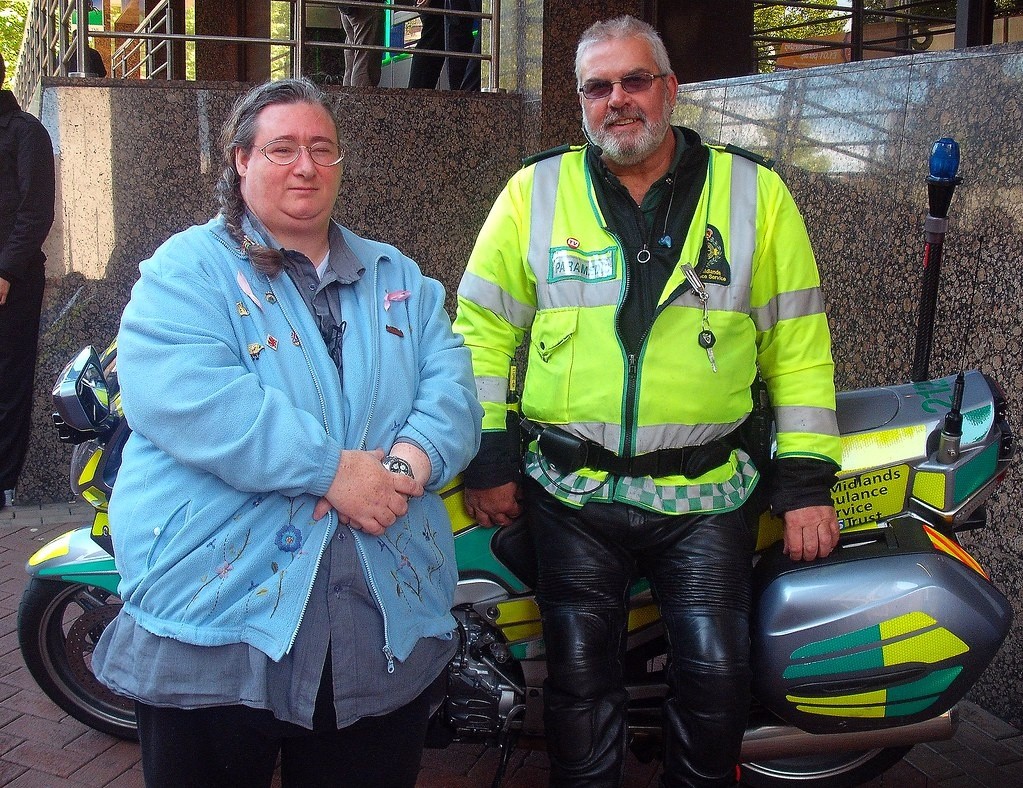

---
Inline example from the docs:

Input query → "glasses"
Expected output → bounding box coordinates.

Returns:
[579,70,665,100]
[252,138,347,166]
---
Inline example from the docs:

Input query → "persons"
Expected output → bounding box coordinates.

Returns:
[336,1,388,87]
[458,30,481,92]
[408,1,473,92]
[90,78,484,788]
[0,51,55,512]
[452,15,841,787]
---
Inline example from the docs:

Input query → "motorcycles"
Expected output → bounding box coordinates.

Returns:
[15,325,1008,787]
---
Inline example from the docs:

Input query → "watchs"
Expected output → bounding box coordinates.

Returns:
[379,454,417,504]
